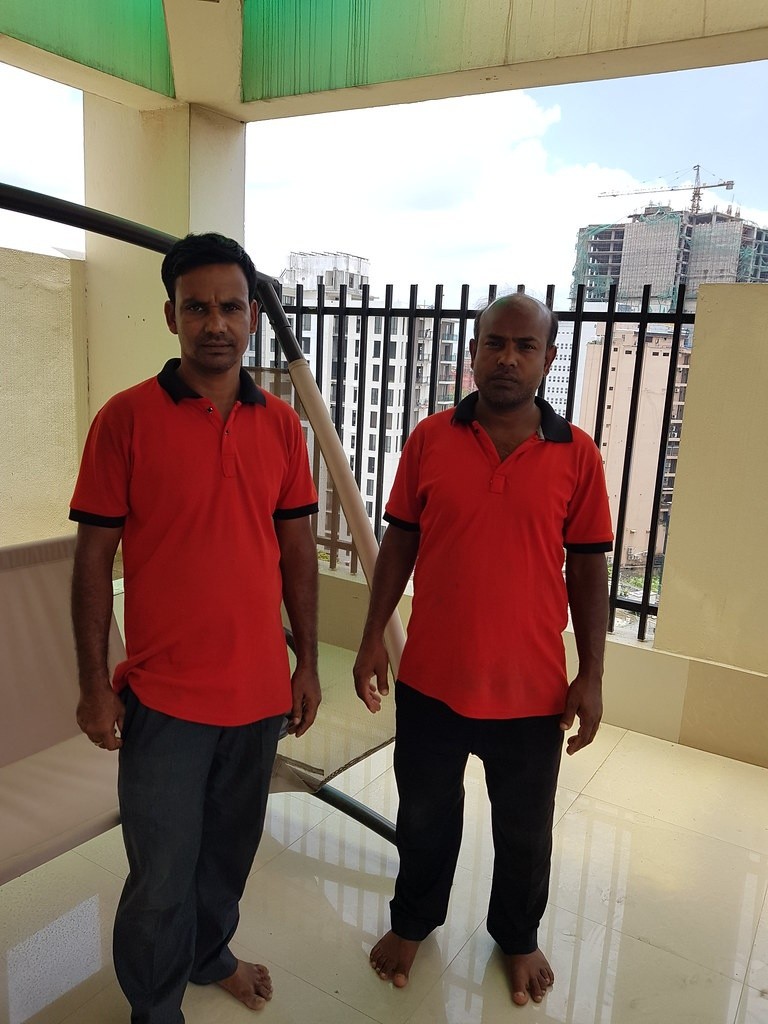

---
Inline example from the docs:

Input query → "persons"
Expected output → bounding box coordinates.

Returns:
[68,234,323,1024]
[353,295,615,1005]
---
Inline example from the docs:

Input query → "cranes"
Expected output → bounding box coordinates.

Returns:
[596,163,734,216]
